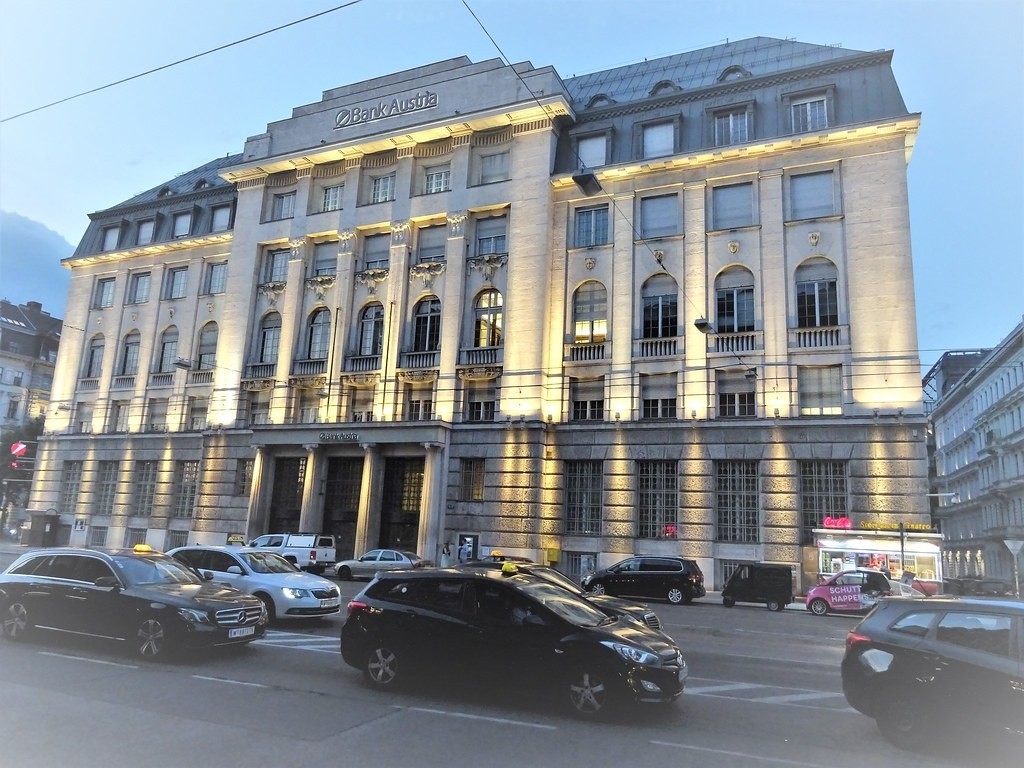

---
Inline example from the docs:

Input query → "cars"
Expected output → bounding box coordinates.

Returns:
[0,544,271,662]
[444,551,664,634]
[581,555,706,603]
[720,562,795,612]
[333,548,436,576]
[950,578,1014,601]
[806,568,893,615]
[338,562,688,715]
[156,544,341,626]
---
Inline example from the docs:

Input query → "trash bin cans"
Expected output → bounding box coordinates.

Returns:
[19,525,30,544]
[30,515,60,547]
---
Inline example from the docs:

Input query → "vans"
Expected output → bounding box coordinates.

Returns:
[839,595,1024,760]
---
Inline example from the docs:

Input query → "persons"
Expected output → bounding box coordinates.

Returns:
[879,561,891,580]
[441,540,451,568]
[460,539,469,563]
[510,589,534,626]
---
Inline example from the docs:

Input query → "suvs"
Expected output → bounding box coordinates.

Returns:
[243,532,337,576]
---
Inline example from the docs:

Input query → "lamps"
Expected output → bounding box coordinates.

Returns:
[507,415,512,421]
[898,407,905,417]
[363,447,369,452]
[425,444,431,452]
[691,410,697,420]
[874,408,880,419]
[614,411,621,421]
[926,492,959,504]
[306,448,312,454]
[520,414,525,422]
[774,409,781,420]
[547,414,552,424]
[927,429,932,436]
